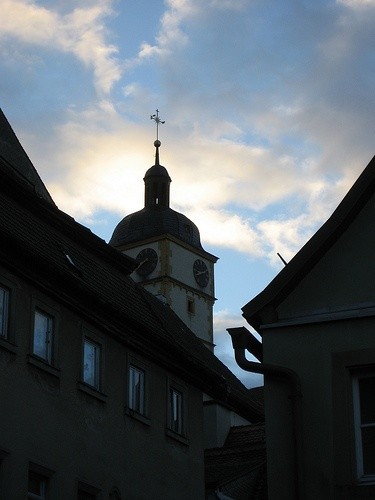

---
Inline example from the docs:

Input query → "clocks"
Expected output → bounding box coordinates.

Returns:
[193,259,209,288]
[135,248,158,275]
